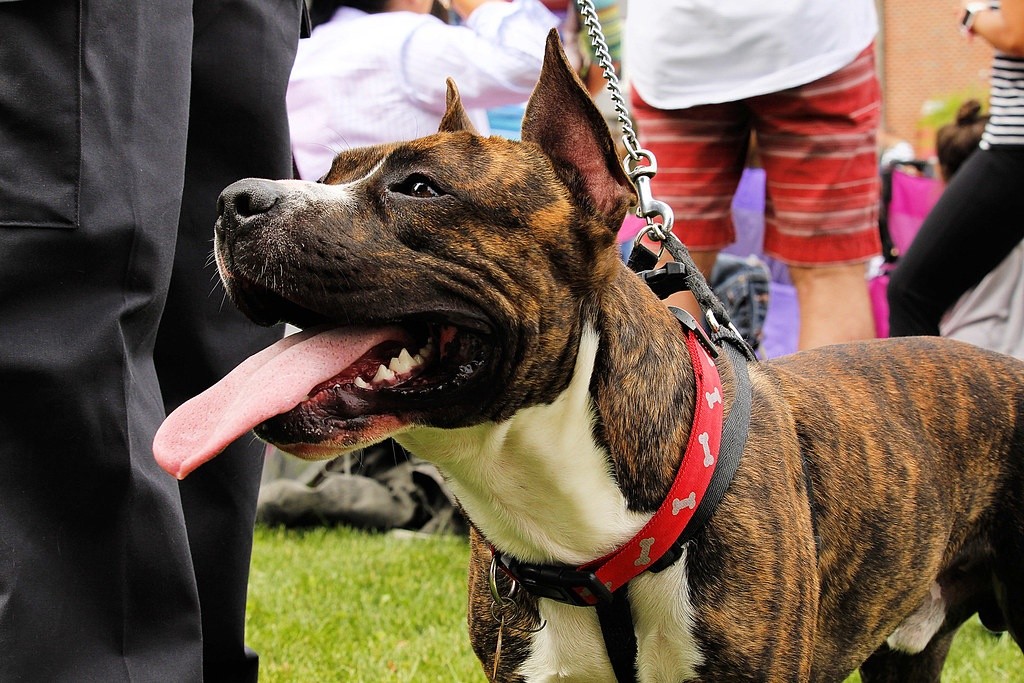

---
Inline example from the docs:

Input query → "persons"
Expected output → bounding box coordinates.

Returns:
[288,0,1024,367]
[0,0,302,683]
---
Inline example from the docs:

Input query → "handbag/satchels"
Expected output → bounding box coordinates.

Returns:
[868,157,952,339]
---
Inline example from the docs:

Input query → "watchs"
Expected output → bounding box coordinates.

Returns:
[961,3,984,29]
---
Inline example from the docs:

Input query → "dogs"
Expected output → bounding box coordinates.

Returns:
[151,25,1024,682]
